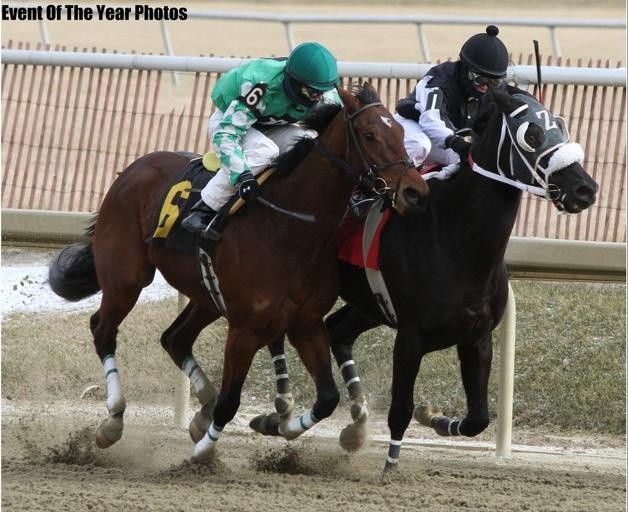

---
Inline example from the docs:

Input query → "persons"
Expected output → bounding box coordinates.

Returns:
[350,26,509,217]
[180,41,344,241]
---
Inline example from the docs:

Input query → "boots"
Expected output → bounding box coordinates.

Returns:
[181,199,221,240]
[350,188,368,224]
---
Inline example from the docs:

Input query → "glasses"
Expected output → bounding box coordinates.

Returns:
[301,87,323,97]
[468,71,503,89]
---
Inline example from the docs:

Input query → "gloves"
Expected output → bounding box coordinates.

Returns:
[239,173,258,201]
[444,135,472,153]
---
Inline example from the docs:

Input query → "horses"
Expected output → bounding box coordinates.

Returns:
[47,81,431,463]
[265,82,600,483]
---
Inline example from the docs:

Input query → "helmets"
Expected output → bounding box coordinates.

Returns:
[459,25,508,78]
[285,42,341,91]
